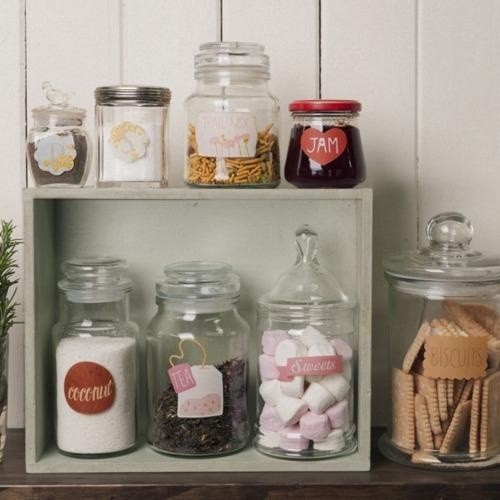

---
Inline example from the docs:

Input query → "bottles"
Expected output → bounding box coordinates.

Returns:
[142,260,256,459]
[51,252,140,460]
[379,211,500,473]
[92,83,173,191]
[26,102,93,191]
[252,224,360,463]
[182,38,283,190]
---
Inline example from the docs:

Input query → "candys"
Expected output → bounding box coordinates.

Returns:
[257,325,353,454]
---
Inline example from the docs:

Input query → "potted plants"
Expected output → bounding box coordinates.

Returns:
[1,218,27,461]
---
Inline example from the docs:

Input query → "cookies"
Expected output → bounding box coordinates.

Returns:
[390,300,500,465]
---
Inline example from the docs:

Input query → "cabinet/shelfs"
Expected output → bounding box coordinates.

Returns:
[22,183,377,475]
